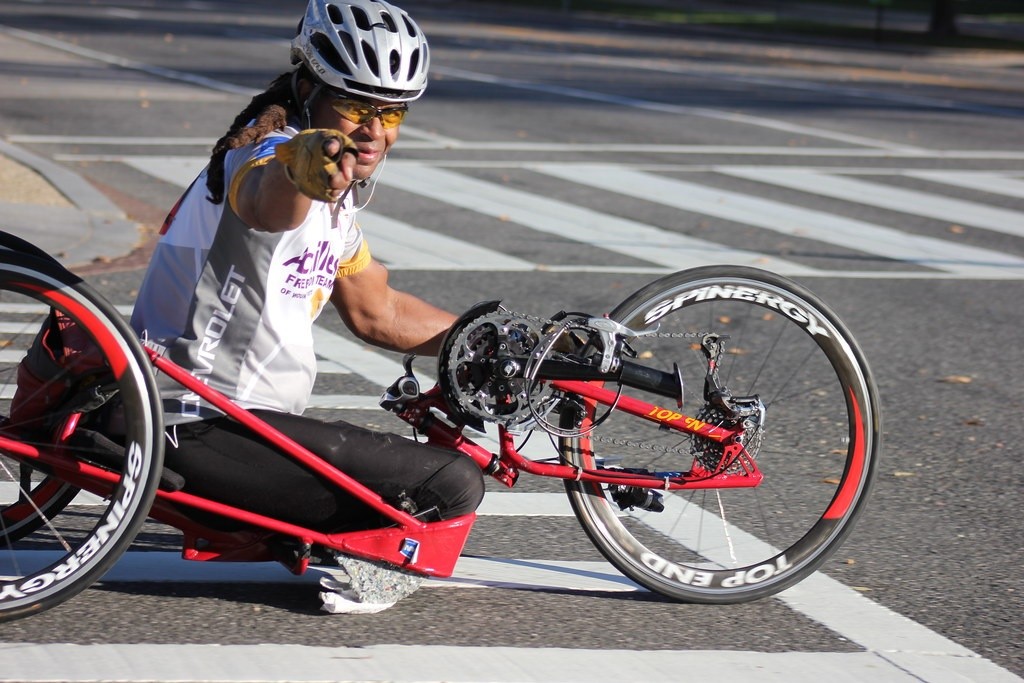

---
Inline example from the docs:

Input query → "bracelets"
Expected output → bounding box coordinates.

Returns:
[284,164,296,184]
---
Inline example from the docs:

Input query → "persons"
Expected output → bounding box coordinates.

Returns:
[130,0,585,545]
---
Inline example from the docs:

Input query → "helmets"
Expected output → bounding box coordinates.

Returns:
[290,0,430,102]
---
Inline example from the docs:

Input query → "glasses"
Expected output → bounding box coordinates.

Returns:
[310,79,408,128]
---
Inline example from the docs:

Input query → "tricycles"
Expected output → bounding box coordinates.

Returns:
[0,230,885,626]
